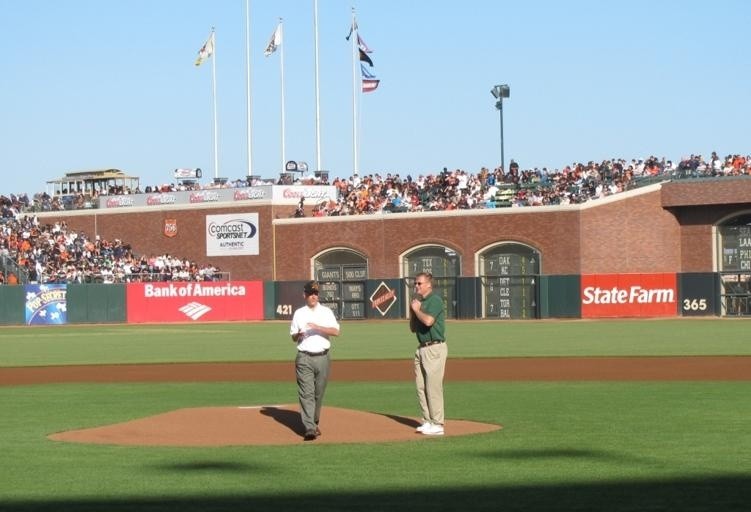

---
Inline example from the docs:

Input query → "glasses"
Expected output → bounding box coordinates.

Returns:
[414,282,425,286]
[309,292,318,295]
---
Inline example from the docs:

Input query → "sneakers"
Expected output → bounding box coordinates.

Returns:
[305,427,321,439]
[416,423,444,435]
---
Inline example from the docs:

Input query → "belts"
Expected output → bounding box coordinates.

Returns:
[302,351,328,356]
[422,340,440,346]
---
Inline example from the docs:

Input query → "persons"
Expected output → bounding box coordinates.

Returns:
[410,273,448,436]
[290,280,340,439]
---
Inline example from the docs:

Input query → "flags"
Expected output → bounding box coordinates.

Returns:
[264,23,282,57]
[195,31,214,65]
[346,13,380,94]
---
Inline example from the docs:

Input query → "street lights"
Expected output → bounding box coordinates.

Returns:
[489,82,513,175]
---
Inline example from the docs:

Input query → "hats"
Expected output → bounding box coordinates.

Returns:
[304,281,320,293]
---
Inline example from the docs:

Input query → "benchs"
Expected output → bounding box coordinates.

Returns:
[381,159,661,215]
[653,161,751,179]
